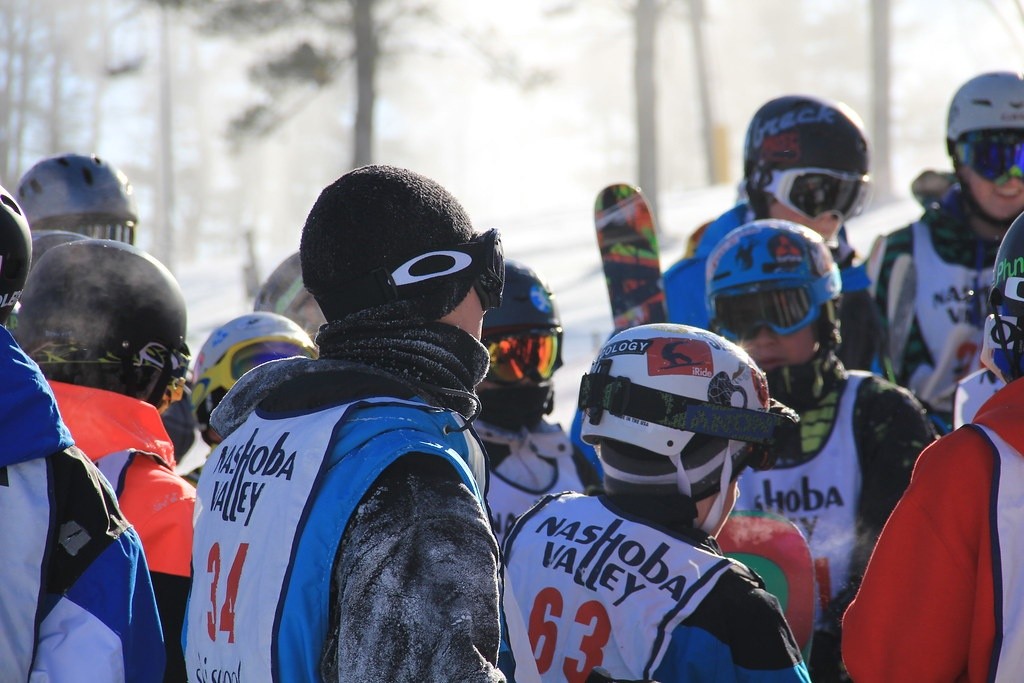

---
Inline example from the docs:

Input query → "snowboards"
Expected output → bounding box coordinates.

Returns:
[591,179,669,330]
[716,508,816,668]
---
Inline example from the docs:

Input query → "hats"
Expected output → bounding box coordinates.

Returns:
[301,165,475,318]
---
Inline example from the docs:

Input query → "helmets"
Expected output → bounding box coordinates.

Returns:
[742,94,867,175]
[579,323,800,504]
[9,238,188,400]
[988,211,1024,303]
[0,185,33,324]
[189,310,318,381]
[31,229,91,272]
[947,72,1024,141]
[705,219,840,322]
[483,261,556,328]
[14,155,138,246]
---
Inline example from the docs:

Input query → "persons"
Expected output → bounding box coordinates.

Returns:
[659,93,892,380]
[0,149,930,683]
[860,72,1024,433]
[838,216,1024,683]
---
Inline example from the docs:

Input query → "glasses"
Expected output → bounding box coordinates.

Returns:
[387,228,505,309]
[949,132,1024,185]
[709,275,819,338]
[482,329,562,381]
[188,337,318,412]
[769,398,800,454]
[164,340,192,385]
[764,168,876,220]
